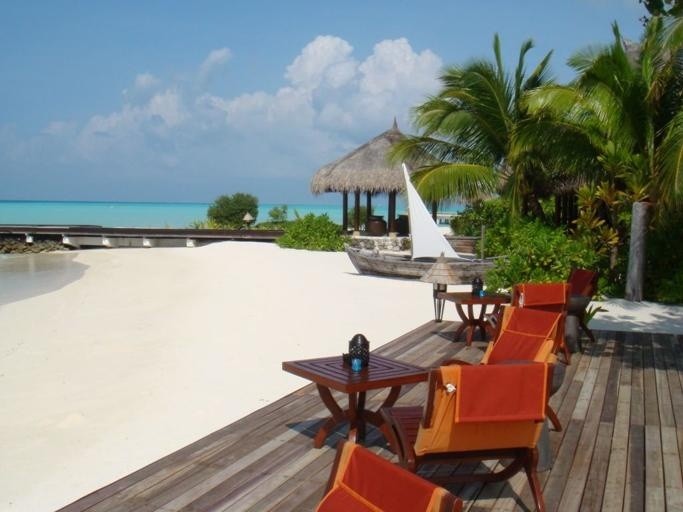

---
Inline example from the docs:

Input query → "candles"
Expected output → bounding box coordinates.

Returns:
[351,358,362,372]
[479,290,484,297]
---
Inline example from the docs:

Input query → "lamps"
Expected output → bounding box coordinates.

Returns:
[419,252,462,323]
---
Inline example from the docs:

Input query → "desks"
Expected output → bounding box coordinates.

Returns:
[282,353,429,448]
[437,292,512,346]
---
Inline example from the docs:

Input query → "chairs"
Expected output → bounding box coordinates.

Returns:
[480,284,572,365]
[316,439,463,512]
[379,363,555,512]
[567,268,600,353]
[440,305,567,431]
